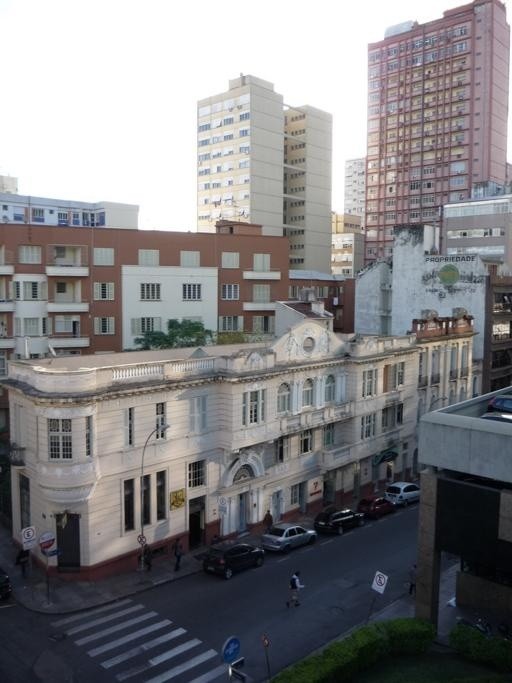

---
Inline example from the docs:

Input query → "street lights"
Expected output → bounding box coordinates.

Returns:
[138,422,173,570]
[24,332,31,360]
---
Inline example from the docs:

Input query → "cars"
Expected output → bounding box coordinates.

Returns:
[359,495,395,519]
[482,410,512,431]
[204,541,264,578]
[383,481,419,509]
[263,521,318,553]
[0,569,15,601]
[488,395,512,413]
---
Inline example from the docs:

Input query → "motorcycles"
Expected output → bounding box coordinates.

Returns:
[456,616,490,641]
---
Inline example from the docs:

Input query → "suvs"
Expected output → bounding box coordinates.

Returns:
[311,506,366,537]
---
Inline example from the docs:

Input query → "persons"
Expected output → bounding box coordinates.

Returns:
[210,534,219,545]
[262,509,273,534]
[286,571,306,608]
[174,537,186,571]
[138,544,152,570]
[408,563,417,594]
